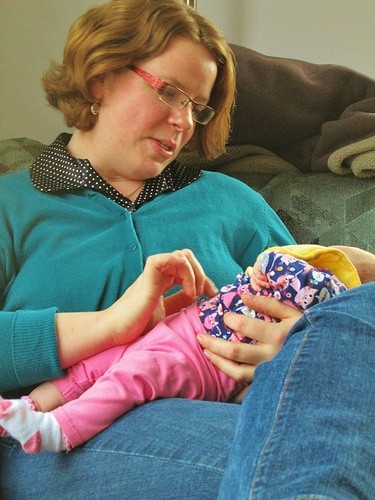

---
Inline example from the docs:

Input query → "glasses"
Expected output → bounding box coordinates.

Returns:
[128,65,217,127]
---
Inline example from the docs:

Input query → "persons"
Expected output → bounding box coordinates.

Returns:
[0,244,375,454]
[0,0,375,500]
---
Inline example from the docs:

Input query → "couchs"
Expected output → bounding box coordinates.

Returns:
[1,138,375,256]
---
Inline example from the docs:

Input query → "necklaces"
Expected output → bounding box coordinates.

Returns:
[126,181,146,200]
